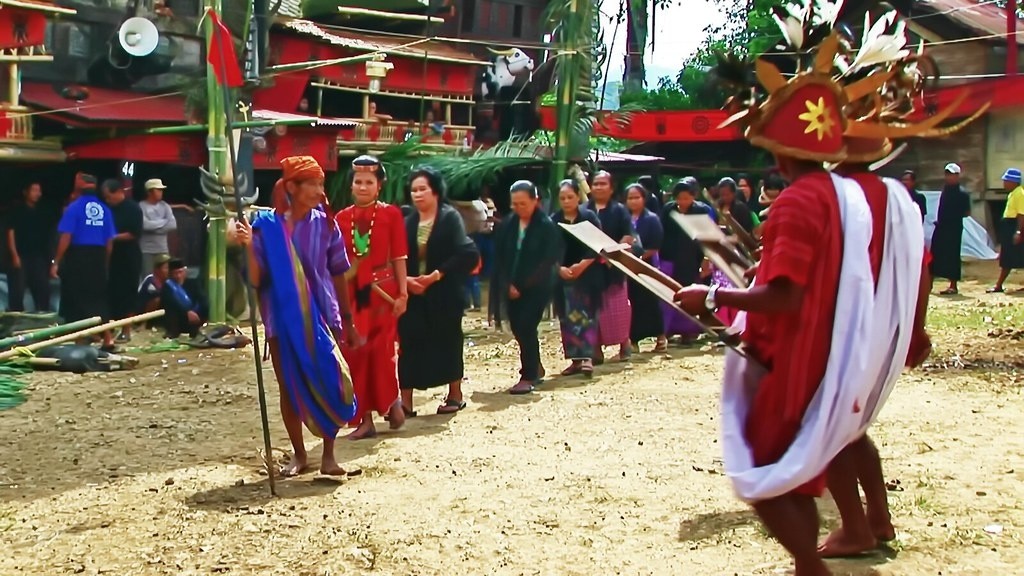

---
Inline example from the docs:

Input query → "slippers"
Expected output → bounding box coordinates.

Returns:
[437,399,466,413]
[384,404,418,420]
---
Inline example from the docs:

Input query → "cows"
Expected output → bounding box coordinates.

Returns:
[481,47,533,101]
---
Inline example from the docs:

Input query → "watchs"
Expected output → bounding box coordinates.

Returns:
[705,284,720,313]
[51,259,59,266]
[1014,230,1022,235]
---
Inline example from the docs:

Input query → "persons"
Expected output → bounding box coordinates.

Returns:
[985,168,1024,293]
[549,170,790,378]
[902,163,971,295]
[8,170,210,351]
[332,154,409,441]
[487,181,564,394]
[385,166,483,421]
[236,156,365,478]
[673,82,932,576]
[463,236,503,331]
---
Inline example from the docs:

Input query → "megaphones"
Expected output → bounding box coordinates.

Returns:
[117,16,159,57]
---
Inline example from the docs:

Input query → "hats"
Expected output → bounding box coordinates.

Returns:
[145,179,167,189]
[944,162,961,173]
[1001,168,1021,183]
[153,253,170,264]
[833,8,992,163]
[746,0,969,163]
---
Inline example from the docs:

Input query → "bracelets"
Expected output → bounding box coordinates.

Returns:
[341,312,353,320]
[639,255,643,260]
[399,292,408,299]
[435,270,441,281]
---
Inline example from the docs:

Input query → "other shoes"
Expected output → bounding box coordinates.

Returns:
[581,361,593,376]
[985,287,1004,293]
[562,366,580,375]
[619,348,631,360]
[655,339,668,352]
[940,288,959,294]
[116,332,131,344]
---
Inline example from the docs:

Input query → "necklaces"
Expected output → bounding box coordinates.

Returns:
[350,200,377,256]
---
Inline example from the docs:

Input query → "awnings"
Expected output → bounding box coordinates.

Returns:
[60,129,355,173]
